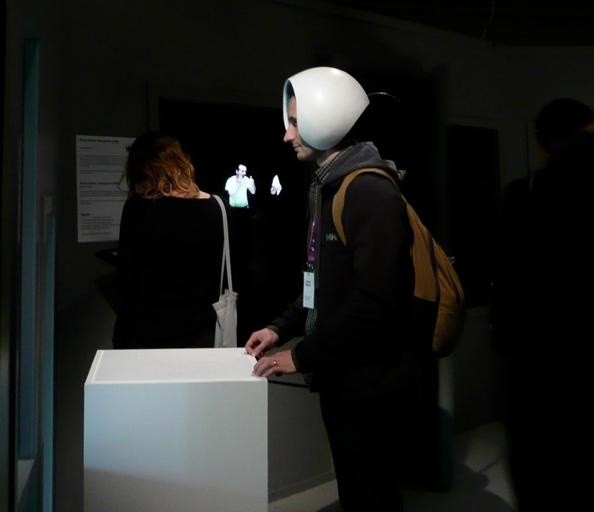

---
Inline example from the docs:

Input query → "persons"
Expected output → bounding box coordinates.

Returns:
[244,64,466,511]
[488,95,593,511]
[224,163,256,212]
[103,132,238,347]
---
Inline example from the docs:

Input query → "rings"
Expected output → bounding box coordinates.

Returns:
[273,358,278,367]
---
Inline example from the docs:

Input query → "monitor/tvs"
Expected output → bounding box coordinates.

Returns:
[146,79,313,246]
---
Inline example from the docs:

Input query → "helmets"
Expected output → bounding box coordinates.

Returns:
[281,65,371,152]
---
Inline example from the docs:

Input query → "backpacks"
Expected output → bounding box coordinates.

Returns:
[331,162,469,357]
[211,286,240,347]
